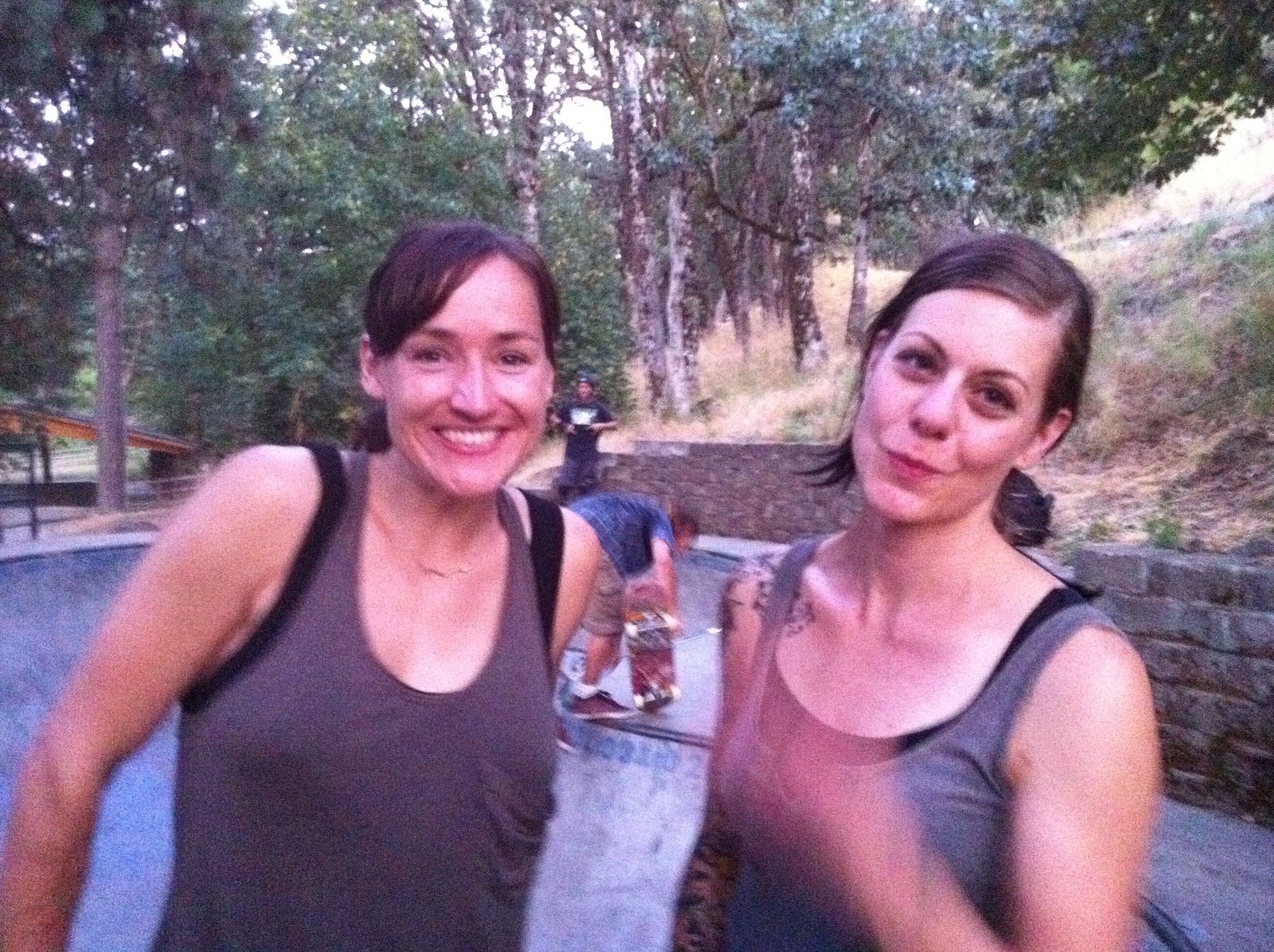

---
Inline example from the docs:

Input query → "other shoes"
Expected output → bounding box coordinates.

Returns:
[557,719,574,752]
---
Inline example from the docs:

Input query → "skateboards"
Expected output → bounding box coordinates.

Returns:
[624,578,681,712]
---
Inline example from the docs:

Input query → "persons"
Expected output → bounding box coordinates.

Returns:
[672,238,1162,952]
[553,494,700,753]
[550,376,620,503]
[0,224,600,952]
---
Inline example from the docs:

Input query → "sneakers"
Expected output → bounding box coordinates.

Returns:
[572,692,633,719]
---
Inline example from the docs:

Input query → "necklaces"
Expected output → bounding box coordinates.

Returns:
[370,506,474,576]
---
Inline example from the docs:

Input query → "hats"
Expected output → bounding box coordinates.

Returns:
[577,376,594,387]
